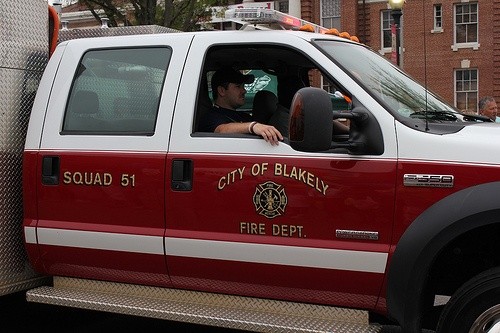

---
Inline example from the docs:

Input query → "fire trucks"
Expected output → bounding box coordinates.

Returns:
[0,0,500,333]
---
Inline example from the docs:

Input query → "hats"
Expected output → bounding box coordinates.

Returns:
[211,68,255,91]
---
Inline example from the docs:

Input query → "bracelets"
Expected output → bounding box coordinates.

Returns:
[249,120,258,134]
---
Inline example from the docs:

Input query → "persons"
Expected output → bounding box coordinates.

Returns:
[269,75,350,135]
[201,68,283,145]
[476,96,500,123]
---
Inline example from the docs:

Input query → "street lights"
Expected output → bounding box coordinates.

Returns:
[389,0,404,66]
